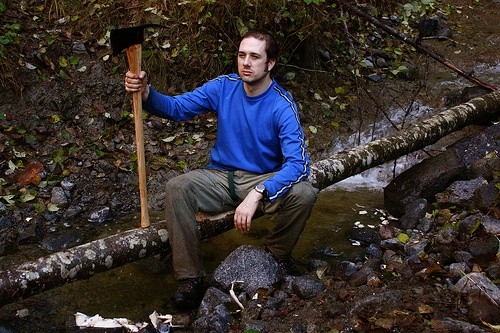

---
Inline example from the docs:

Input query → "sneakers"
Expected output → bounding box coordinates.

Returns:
[174,277,206,310]
[266,246,303,277]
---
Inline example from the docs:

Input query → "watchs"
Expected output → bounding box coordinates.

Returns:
[255,183,269,201]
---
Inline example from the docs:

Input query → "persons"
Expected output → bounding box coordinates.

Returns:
[125,28,317,306]
